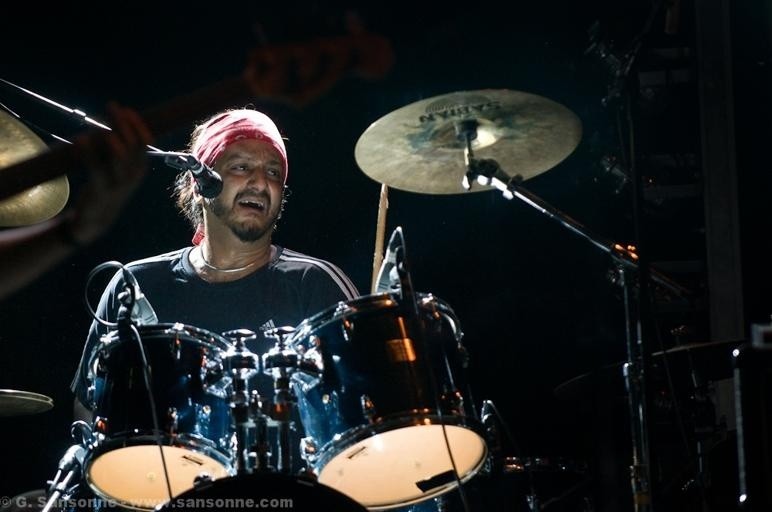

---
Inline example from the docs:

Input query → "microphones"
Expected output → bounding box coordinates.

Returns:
[187,154,224,198]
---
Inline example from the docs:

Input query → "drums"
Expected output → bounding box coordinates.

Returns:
[81,322,237,508]
[289,288,491,510]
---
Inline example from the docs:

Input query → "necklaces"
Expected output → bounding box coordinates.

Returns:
[200,238,271,273]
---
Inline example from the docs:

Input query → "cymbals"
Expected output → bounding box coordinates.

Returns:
[1,387,54,417]
[355,91,584,196]
[2,108,70,228]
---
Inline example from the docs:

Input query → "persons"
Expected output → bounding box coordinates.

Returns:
[69,110,445,512]
[0,101,154,302]
